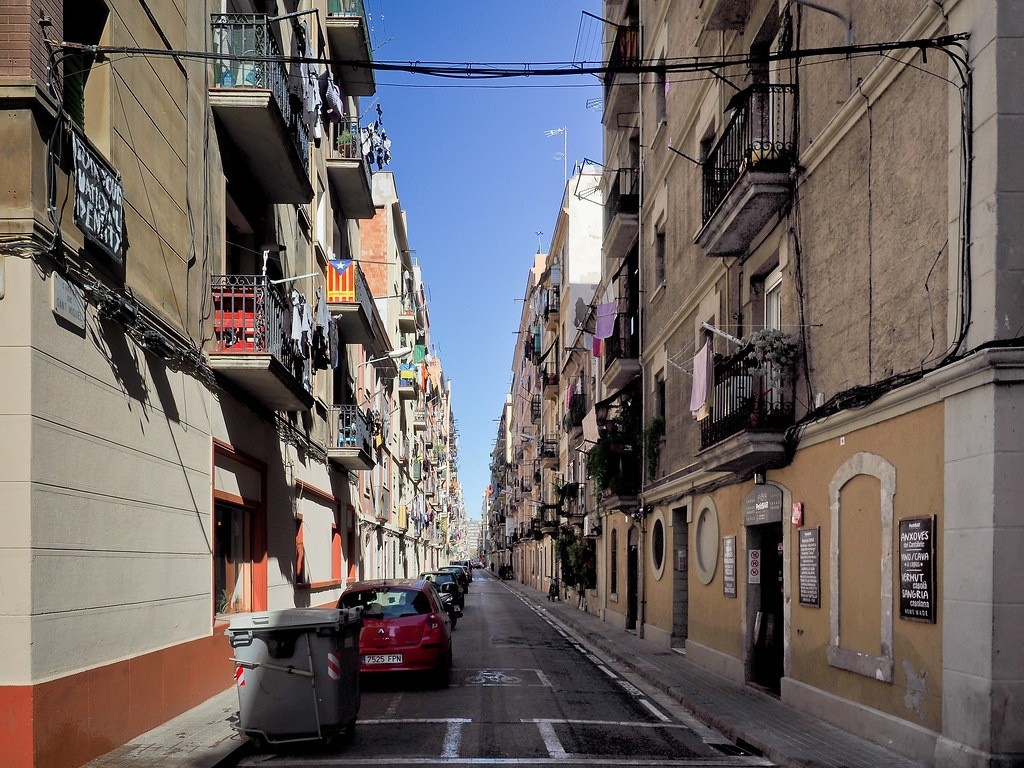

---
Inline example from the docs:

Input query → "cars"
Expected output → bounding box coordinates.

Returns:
[462,566,469,584]
[472,562,482,569]
[332,580,454,689]
[436,565,469,595]
[419,571,465,610]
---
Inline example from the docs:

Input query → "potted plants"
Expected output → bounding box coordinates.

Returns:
[568,544,594,585]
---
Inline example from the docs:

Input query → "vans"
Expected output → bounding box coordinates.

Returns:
[450,559,473,583]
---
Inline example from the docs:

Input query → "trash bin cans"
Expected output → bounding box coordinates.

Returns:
[223,606,365,754]
